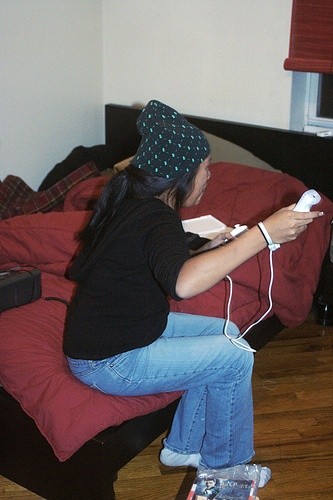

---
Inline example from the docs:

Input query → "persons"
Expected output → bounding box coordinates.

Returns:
[61,99,325,488]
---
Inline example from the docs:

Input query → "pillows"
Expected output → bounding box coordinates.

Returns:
[196,126,282,174]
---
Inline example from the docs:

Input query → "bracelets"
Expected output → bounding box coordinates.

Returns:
[256,221,273,246]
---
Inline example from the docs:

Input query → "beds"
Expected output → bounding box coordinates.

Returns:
[0,103,332,500]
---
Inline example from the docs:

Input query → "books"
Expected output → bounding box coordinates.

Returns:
[180,213,227,237]
[185,473,256,500]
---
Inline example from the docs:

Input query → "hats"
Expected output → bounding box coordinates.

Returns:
[129,99,212,180]
